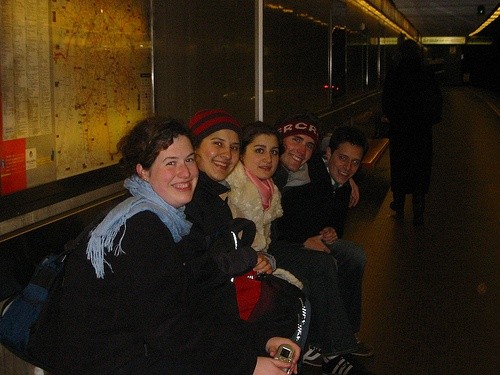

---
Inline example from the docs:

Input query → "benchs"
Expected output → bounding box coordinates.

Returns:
[359,136,390,167]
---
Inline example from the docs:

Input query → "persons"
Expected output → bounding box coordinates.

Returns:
[184,108,311,359]
[219,120,305,289]
[31,115,301,375]
[272,113,369,375]
[381,39,441,226]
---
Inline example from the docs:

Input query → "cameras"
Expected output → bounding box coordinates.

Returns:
[272,343,294,374]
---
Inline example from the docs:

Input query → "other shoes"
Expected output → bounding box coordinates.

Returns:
[390,201,404,212]
[413,216,424,225]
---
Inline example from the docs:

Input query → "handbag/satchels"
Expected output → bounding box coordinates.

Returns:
[0,193,129,375]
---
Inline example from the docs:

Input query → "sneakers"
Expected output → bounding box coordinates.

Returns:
[303,344,325,368]
[321,354,369,375]
[351,333,374,357]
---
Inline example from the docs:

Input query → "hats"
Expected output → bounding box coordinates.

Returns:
[189,107,243,149]
[273,111,320,150]
